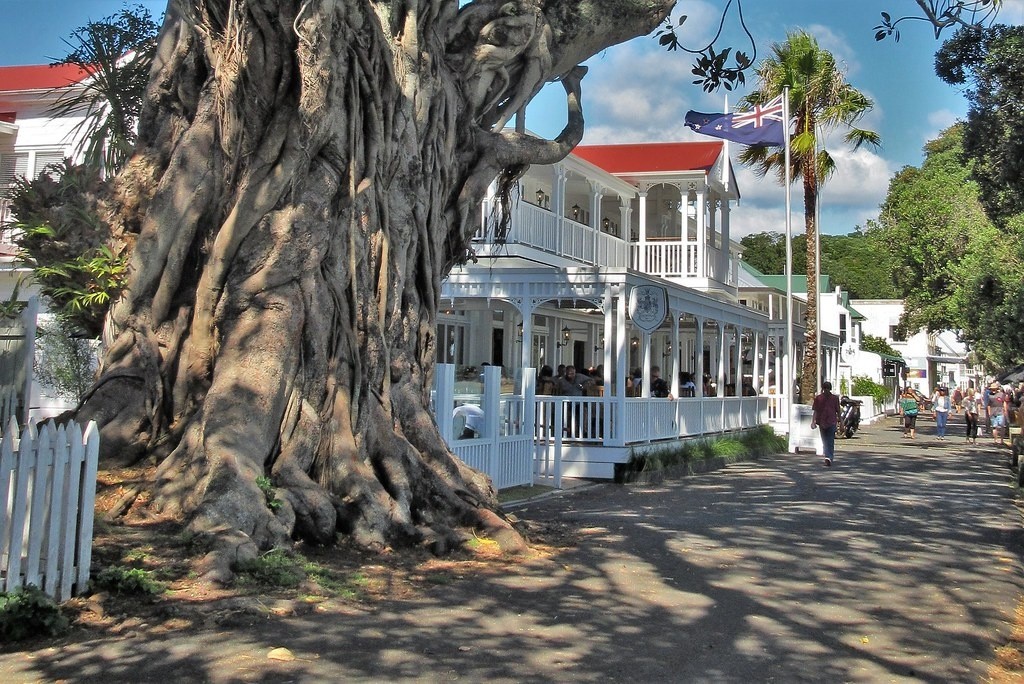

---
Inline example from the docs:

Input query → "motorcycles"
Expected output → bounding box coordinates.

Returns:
[834,395,865,439]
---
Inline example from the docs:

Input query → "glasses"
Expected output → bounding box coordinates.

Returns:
[652,375,658,378]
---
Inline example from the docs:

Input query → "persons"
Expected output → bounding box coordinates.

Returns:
[535,364,757,442]
[898,380,1024,445]
[811,381,844,466]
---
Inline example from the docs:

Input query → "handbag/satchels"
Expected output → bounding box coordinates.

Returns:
[977,427,982,436]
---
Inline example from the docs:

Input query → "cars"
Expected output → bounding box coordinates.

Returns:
[900,388,933,410]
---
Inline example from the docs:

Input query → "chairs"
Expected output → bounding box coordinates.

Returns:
[535,377,751,445]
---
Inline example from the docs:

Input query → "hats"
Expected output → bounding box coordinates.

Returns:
[989,384,997,388]
[989,371,992,373]
[940,387,945,391]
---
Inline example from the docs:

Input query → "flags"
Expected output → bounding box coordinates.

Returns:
[684,94,784,143]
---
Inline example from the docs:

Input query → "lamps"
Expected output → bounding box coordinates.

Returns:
[630,336,639,353]
[535,188,545,206]
[572,203,581,220]
[595,333,604,352]
[515,320,523,343]
[557,325,571,348]
[602,217,610,232]
[663,341,671,358]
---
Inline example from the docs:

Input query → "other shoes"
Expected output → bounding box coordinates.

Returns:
[911,437,914,439]
[941,438,944,439]
[825,457,831,465]
